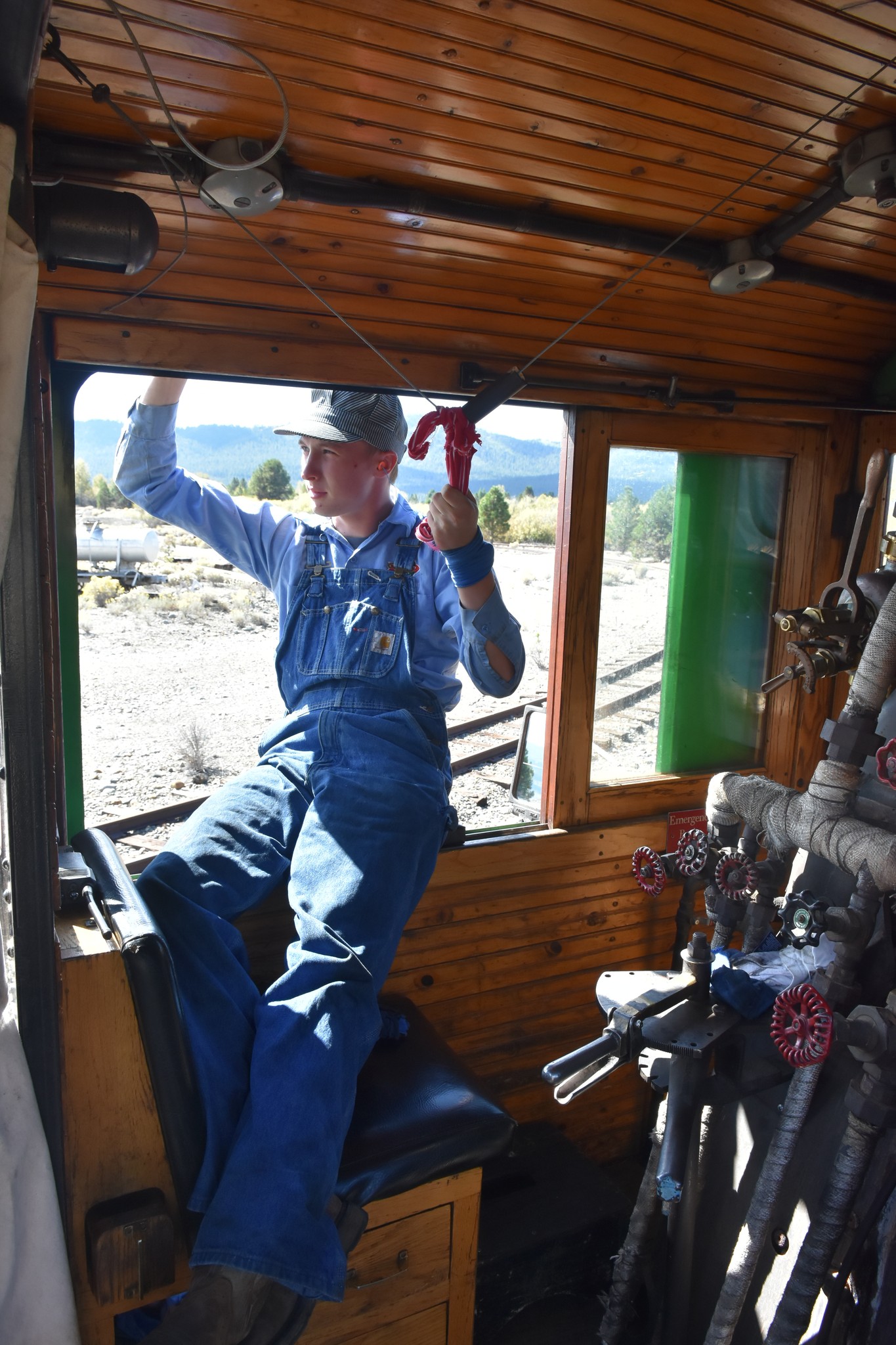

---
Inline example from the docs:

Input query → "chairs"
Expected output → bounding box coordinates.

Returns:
[47,827,513,1345]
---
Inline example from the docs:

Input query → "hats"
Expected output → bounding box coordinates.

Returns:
[273,386,408,463]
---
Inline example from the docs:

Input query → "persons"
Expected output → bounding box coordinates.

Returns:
[117,371,524,1345]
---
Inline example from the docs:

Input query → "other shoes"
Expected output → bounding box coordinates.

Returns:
[147,1262,276,1345]
[242,1189,368,1345]
